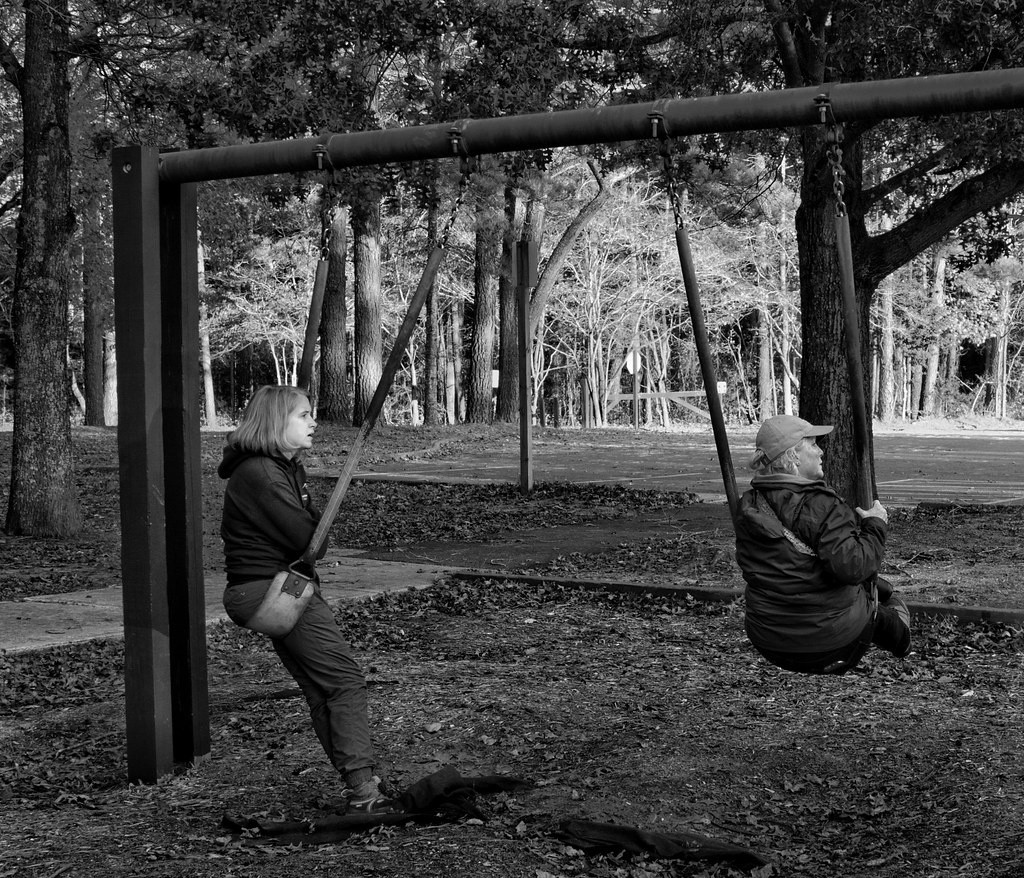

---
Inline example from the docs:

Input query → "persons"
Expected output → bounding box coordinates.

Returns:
[735,415,912,675]
[217,386,396,813]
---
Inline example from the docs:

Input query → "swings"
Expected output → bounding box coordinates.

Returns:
[221,167,476,633]
[646,120,882,677]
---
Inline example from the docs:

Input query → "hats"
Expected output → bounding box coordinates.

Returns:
[755,415,833,465]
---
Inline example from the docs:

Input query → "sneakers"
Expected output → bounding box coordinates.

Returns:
[345,781,414,820]
[886,596,913,658]
